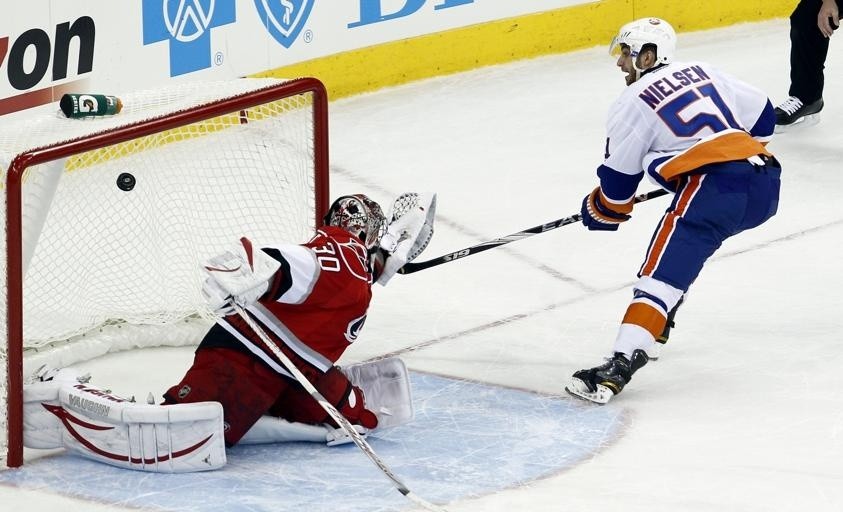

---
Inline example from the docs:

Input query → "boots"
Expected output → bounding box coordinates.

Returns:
[572,349,648,395]
[775,96,824,125]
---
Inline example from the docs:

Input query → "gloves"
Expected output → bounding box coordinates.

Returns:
[581,186,635,231]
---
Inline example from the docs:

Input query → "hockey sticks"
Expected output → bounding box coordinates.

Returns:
[229,299,466,512]
[379,188,670,273]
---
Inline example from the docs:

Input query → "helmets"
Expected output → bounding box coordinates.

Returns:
[608,17,677,64]
[323,194,386,250]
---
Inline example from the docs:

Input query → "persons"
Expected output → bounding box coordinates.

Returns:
[567,13,783,400]
[774,2,842,125]
[11,191,425,482]
